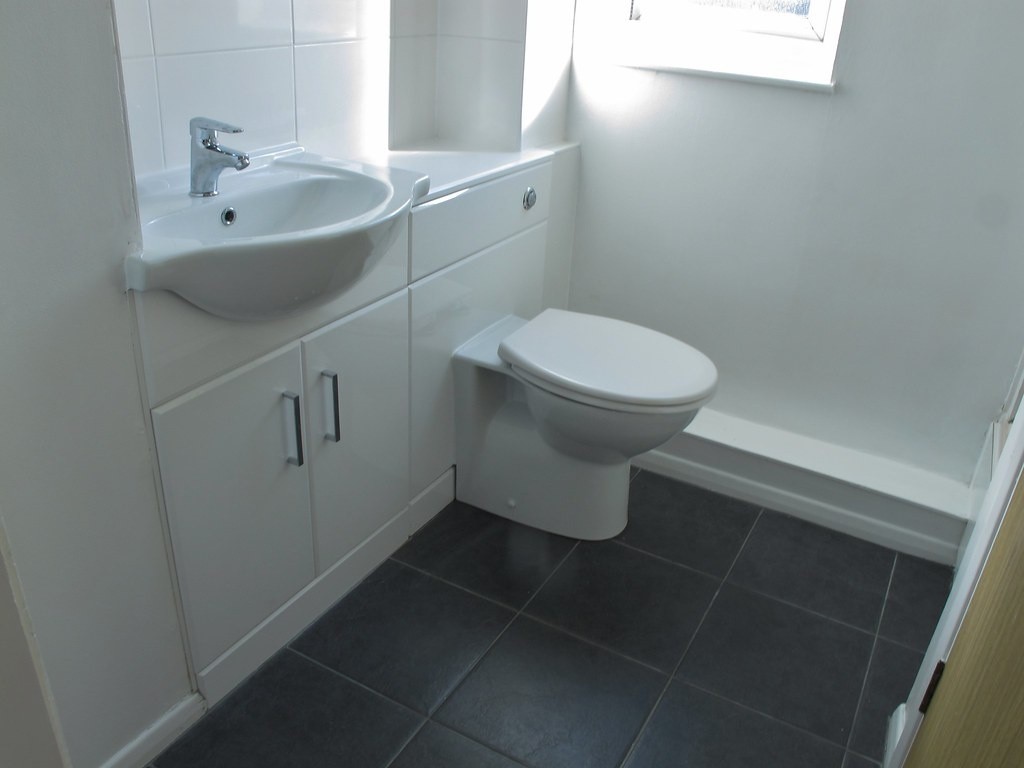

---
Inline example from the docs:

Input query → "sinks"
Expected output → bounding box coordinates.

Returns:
[129,136,430,323]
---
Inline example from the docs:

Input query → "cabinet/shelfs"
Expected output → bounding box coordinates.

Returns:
[134,161,560,708]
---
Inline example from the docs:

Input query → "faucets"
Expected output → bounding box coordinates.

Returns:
[188,116,252,198]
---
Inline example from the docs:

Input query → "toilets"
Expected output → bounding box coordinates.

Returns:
[450,307,721,542]
